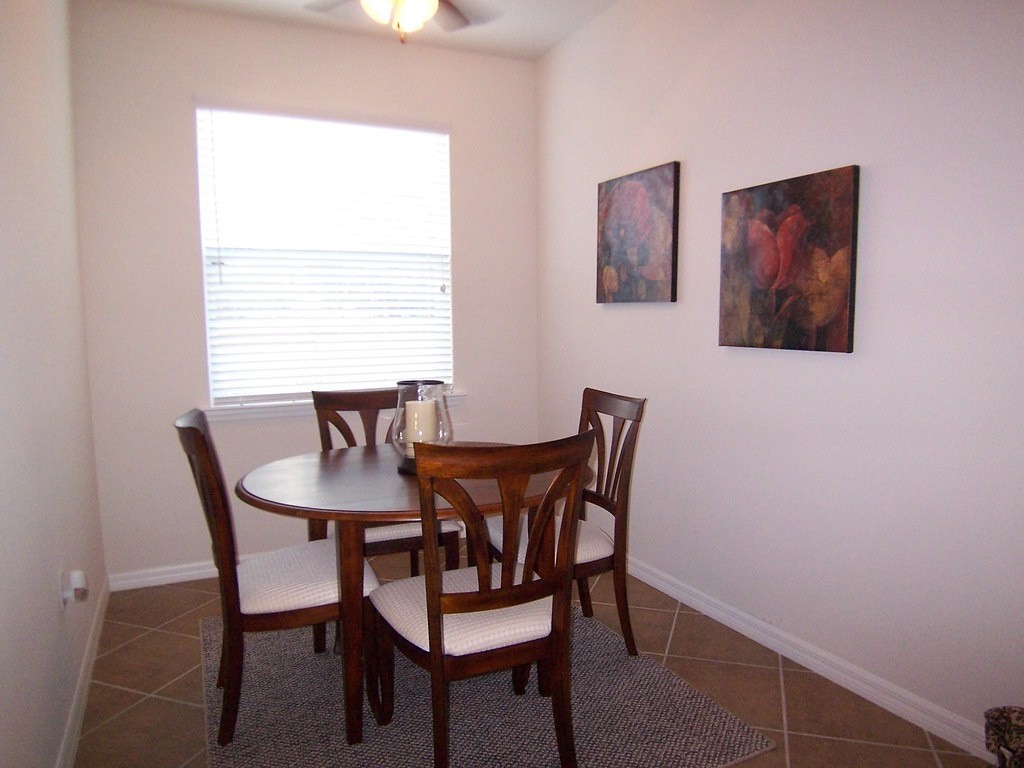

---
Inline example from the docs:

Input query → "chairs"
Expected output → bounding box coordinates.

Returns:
[367,427,598,768]
[172,407,396,747]
[310,388,462,656]
[466,387,647,656]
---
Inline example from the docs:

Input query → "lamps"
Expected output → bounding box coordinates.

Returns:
[356,0,442,45]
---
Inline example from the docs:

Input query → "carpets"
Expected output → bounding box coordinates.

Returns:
[194,592,779,768]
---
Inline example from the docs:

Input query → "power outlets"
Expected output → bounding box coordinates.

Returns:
[60,570,89,609]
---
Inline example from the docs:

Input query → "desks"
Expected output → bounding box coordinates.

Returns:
[234,440,594,746]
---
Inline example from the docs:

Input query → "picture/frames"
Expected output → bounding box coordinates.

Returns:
[718,165,861,353]
[595,158,680,304]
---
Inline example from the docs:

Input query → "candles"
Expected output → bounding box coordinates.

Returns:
[405,400,438,458]
